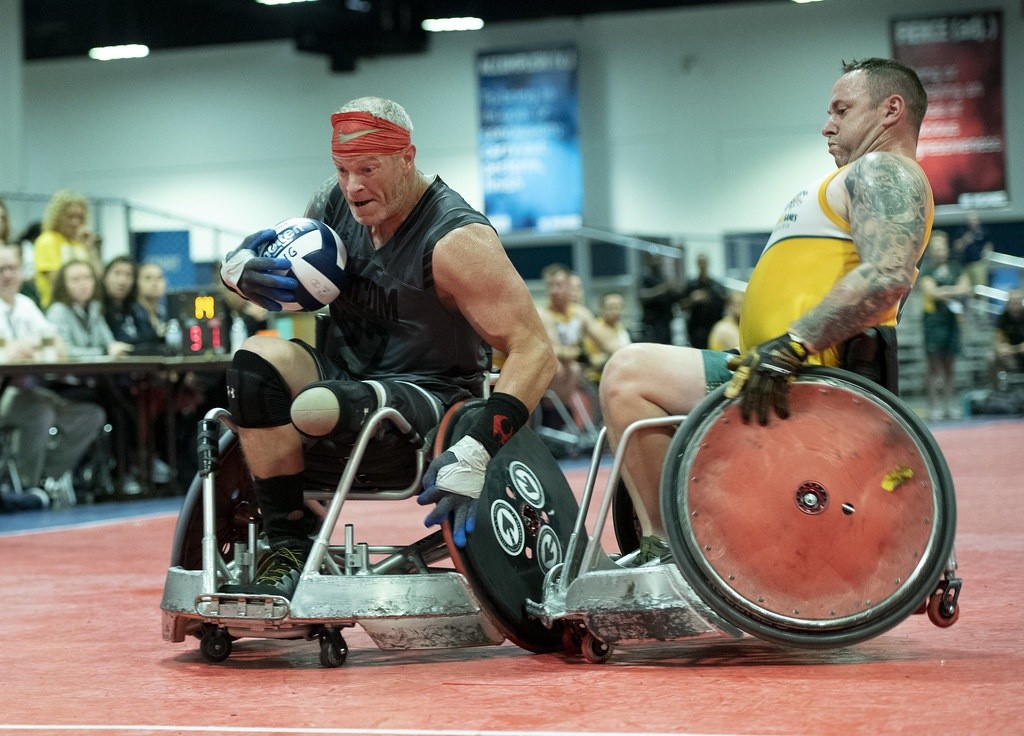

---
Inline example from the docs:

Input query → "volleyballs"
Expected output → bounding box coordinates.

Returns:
[257,216,347,312]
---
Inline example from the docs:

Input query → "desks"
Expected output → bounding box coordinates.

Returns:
[0,354,234,500]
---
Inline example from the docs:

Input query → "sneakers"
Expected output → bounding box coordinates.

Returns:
[249,536,308,604]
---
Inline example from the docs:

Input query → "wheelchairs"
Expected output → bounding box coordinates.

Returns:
[161,371,582,668]
[523,360,962,663]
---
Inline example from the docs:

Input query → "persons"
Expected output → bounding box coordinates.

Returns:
[219,94,559,601]
[918,209,1024,420]
[490,244,740,431]
[0,189,269,515]
[597,57,934,568]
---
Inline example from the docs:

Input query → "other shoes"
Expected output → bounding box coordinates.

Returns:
[0,484,50,512]
[927,409,943,423]
[945,406,962,421]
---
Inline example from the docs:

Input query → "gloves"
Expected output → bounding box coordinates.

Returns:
[417,434,490,548]
[723,330,814,426]
[218,228,298,312]
